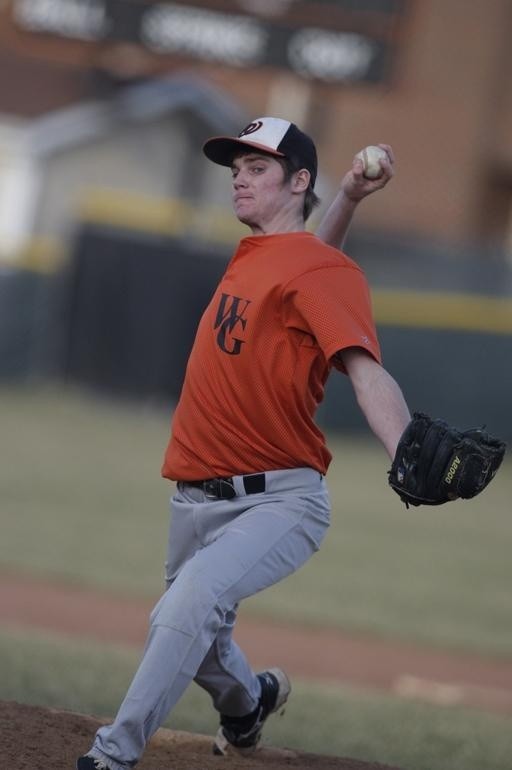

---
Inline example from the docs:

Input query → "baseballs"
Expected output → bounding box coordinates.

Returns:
[356,144,391,179]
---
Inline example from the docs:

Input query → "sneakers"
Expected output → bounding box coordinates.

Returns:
[213,666,290,755]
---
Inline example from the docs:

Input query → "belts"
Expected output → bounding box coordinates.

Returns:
[186,473,265,501]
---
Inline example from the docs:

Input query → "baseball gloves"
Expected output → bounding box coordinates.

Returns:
[390,410,505,508]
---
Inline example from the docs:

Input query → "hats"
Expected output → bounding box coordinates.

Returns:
[202,117,317,183]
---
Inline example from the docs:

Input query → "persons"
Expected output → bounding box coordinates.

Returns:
[73,116,506,769]
